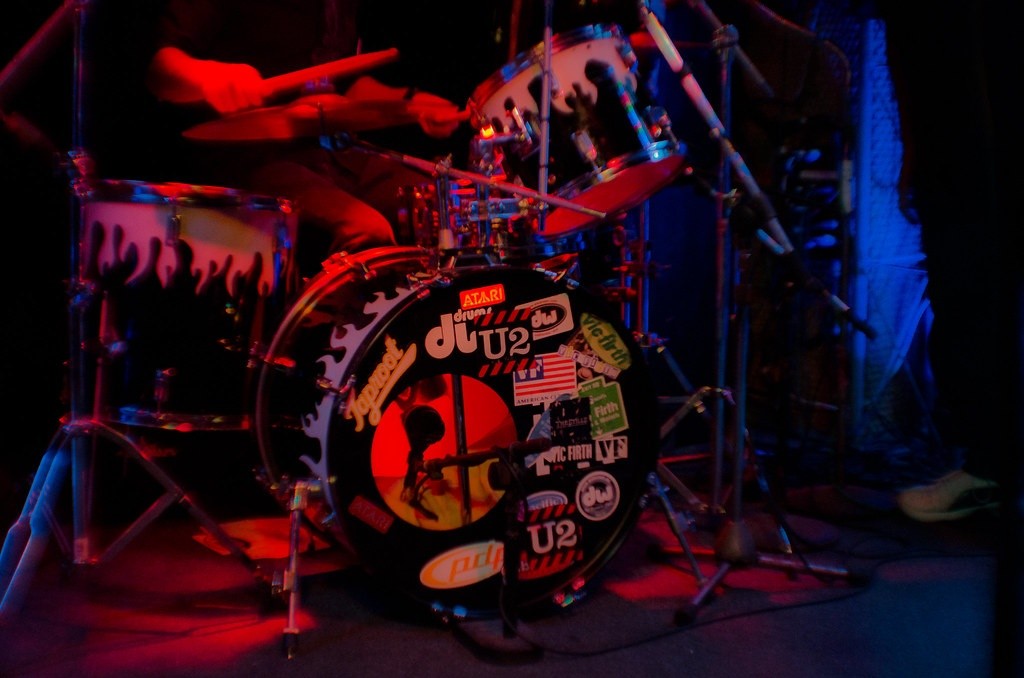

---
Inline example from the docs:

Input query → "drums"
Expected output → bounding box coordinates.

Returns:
[253,242,664,620]
[398,175,583,288]
[67,176,297,434]
[463,22,688,240]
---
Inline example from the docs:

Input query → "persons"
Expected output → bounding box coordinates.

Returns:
[708,0,1024,521]
[144,0,460,336]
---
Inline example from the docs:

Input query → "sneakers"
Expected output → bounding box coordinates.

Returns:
[897,471,1004,521]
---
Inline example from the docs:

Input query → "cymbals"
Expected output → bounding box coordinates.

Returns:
[180,94,418,142]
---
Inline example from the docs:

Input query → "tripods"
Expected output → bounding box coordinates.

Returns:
[0,0,289,639]
[648,1,878,625]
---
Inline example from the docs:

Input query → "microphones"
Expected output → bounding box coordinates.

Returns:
[398,406,445,504]
